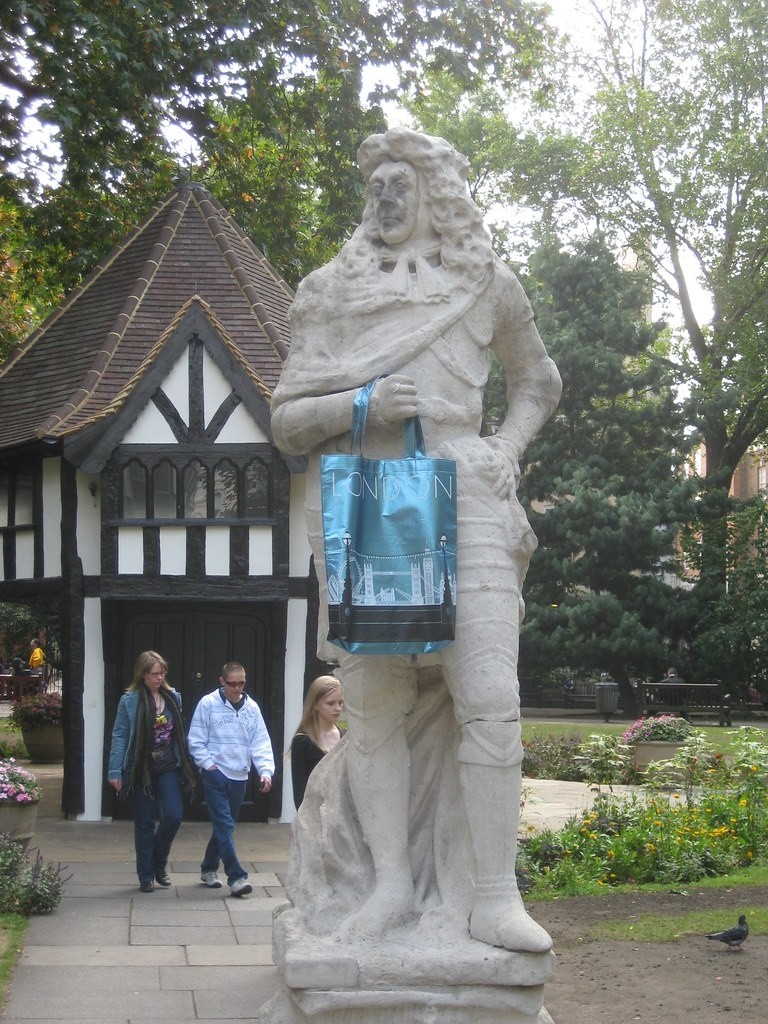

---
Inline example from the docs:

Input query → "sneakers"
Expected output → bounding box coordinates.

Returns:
[230,878,253,897]
[200,870,222,888]
[140,869,172,893]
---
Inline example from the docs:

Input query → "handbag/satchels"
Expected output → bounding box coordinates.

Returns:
[322,375,460,655]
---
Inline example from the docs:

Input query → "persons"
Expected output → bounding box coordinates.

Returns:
[287,675,349,813]
[654,667,693,723]
[263,126,561,957]
[8,639,43,696]
[108,651,184,893]
[188,661,275,897]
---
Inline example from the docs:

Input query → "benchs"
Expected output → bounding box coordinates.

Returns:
[563,680,624,710]
[638,679,736,728]
[0,672,48,703]
[518,675,545,708]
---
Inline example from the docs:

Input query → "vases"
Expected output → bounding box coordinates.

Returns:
[634,741,689,790]
[0,799,39,854]
[22,725,64,764]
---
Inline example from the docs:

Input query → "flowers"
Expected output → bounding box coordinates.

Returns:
[0,756,44,808]
[4,690,63,733]
[623,712,701,746]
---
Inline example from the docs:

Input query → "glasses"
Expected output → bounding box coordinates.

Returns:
[148,670,168,678]
[223,677,246,687]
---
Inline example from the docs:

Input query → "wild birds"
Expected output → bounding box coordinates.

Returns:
[704,914,750,949]
